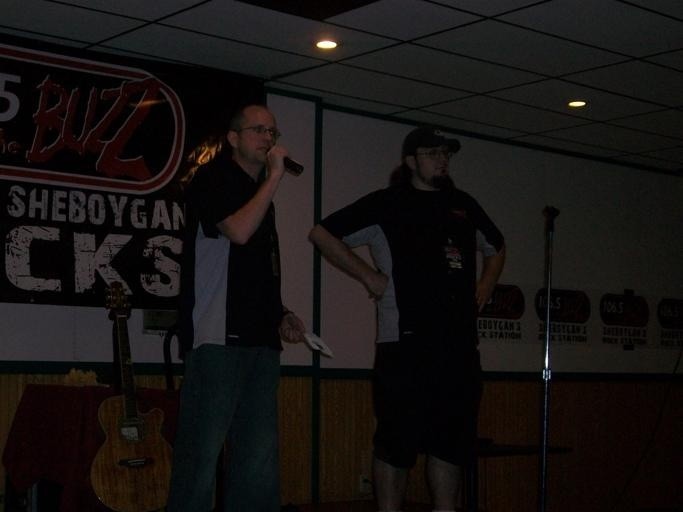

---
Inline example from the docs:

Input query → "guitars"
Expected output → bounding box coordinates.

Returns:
[90,281,173,512]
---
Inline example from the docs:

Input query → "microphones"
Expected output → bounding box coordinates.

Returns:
[282,156,305,176]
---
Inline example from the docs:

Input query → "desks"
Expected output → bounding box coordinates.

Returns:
[0,383,228,512]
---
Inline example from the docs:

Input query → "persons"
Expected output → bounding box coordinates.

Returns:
[166,105,305,512]
[306,126,507,512]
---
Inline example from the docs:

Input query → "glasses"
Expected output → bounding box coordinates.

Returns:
[416,149,452,160]
[237,125,282,140]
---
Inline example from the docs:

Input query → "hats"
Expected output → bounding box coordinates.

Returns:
[402,127,452,156]
